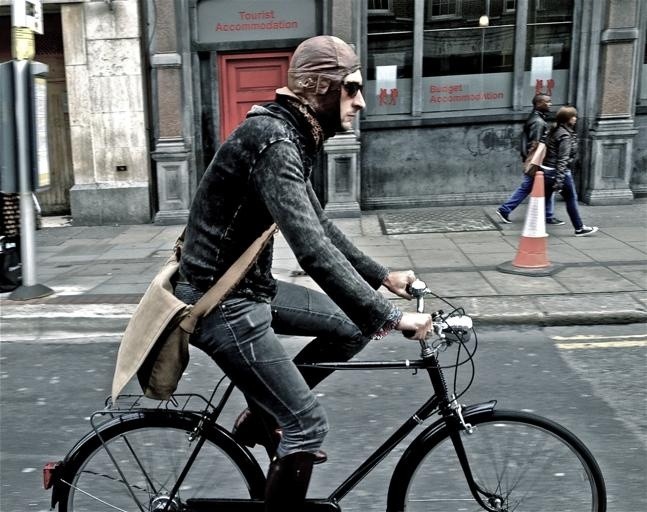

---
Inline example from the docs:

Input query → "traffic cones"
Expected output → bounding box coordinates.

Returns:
[492,171,563,279]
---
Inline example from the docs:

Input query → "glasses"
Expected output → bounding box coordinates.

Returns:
[345,80,362,98]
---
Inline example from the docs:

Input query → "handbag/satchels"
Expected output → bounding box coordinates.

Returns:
[523,128,552,177]
[111,247,197,407]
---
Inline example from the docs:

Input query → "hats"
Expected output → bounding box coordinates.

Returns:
[289,36,362,134]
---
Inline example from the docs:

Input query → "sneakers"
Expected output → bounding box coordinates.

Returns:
[574,225,599,237]
[496,210,512,223]
[546,218,564,225]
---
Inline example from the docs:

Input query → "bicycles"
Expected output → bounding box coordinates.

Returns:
[42,279,608,512]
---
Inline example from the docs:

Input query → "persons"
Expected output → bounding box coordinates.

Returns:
[167,33,434,512]
[544,106,598,237]
[496,94,565,226]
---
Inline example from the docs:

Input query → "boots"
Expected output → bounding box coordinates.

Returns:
[231,403,327,462]
[263,451,315,512]
